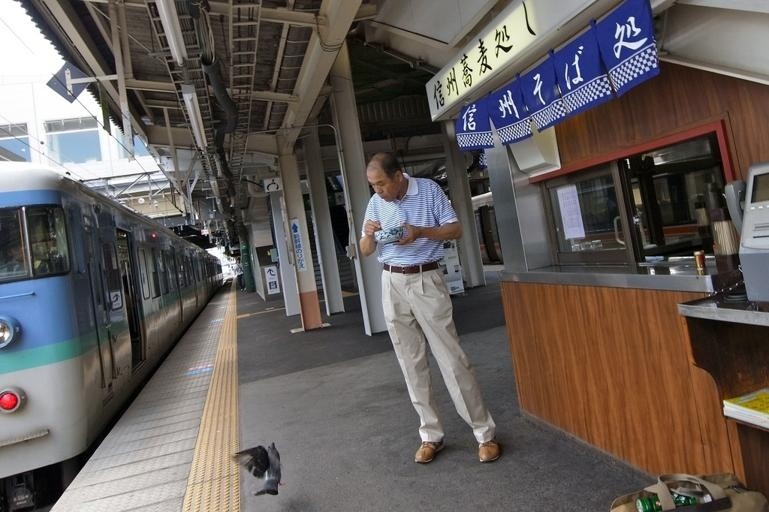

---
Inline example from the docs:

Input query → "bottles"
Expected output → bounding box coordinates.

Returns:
[692,249,706,272]
[635,492,713,512]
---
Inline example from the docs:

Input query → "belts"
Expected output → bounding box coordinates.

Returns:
[383,261,438,274]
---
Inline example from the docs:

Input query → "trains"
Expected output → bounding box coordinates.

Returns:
[449,141,726,265]
[1,164,224,511]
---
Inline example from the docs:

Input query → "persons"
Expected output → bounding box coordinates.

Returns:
[0,230,50,272]
[360,152,501,463]
[231,256,246,291]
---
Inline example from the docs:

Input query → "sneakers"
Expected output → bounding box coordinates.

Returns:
[414,440,444,464]
[478,437,501,464]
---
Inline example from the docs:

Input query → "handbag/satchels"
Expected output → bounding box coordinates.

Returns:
[606,469,769,512]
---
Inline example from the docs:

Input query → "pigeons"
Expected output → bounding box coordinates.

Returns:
[228,442,287,496]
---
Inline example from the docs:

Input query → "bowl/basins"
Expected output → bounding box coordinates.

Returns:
[374,225,404,245]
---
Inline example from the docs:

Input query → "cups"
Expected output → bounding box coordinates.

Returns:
[571,238,603,251]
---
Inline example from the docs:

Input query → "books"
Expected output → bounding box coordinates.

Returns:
[722,386,769,429]
